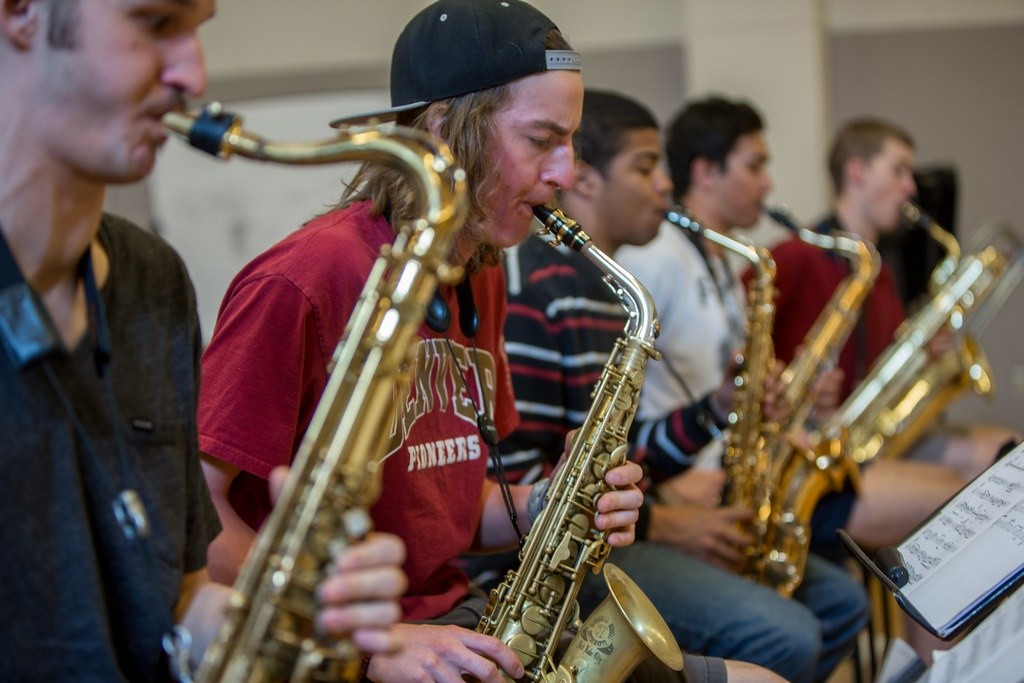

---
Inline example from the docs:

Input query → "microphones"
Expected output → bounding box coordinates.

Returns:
[874,546,909,589]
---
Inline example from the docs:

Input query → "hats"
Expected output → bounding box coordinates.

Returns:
[329,0,581,130]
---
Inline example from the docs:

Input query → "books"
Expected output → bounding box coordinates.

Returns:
[881,437,1023,683]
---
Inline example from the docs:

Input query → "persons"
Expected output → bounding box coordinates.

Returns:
[196,3,791,683]
[735,107,1024,477]
[484,84,873,683]
[0,0,408,682]
[616,91,979,682]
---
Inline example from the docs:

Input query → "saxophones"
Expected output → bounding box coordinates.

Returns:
[465,186,686,683]
[160,104,474,683]
[668,202,1024,599]
